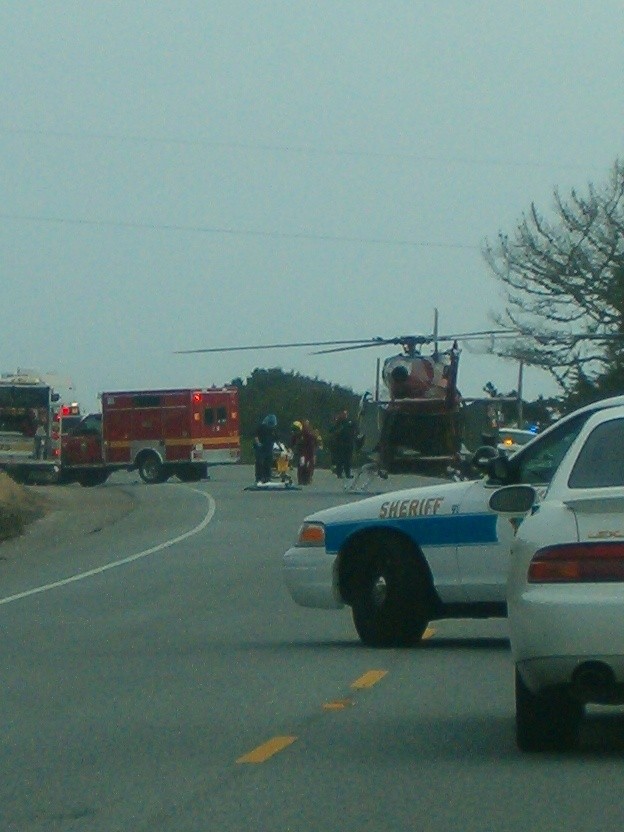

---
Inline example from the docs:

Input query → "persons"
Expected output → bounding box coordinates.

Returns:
[252,409,359,483]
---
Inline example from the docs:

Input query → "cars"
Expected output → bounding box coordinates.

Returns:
[487,405,624,753]
[280,394,624,648]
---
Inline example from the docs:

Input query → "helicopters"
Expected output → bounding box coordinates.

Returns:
[171,329,624,431]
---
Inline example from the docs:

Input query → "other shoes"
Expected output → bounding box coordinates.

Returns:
[257,481,262,487]
[346,475,354,479]
[337,474,343,478]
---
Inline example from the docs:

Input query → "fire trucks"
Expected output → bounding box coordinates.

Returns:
[0,366,61,484]
[58,387,241,487]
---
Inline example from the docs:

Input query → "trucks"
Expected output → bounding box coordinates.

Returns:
[369,409,463,478]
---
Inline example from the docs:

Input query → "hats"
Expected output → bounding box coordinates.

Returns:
[263,414,277,427]
[290,422,303,432]
